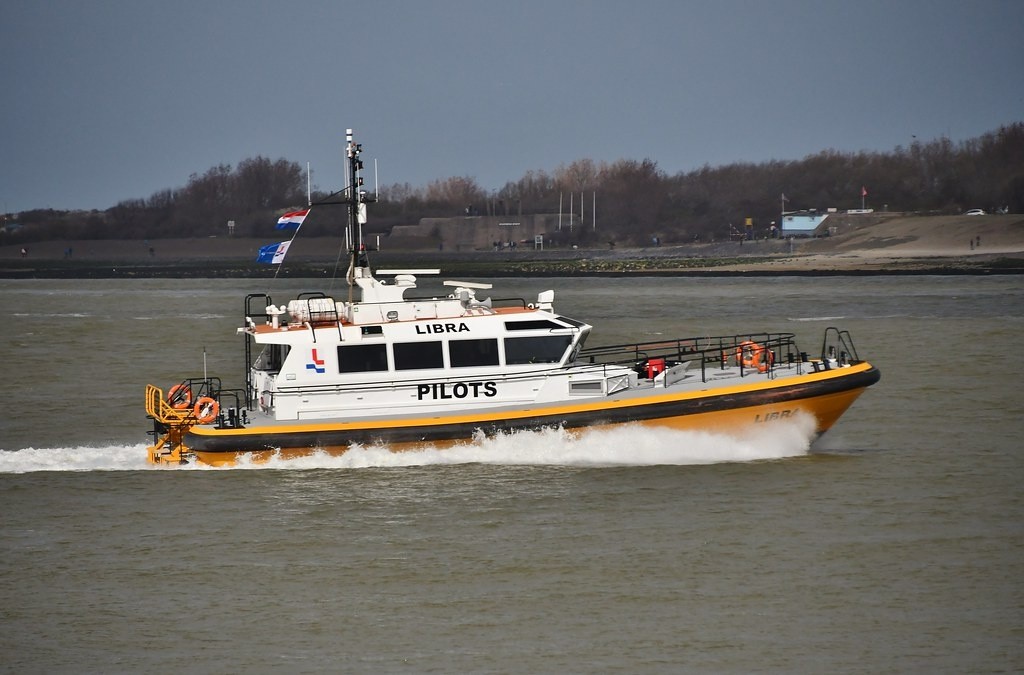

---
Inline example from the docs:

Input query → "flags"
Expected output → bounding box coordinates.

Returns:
[275,208,310,231]
[861,185,867,196]
[257,240,292,264]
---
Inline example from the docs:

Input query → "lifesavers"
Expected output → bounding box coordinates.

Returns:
[193,398,218,422]
[168,385,191,409]
[754,347,773,371]
[736,341,760,366]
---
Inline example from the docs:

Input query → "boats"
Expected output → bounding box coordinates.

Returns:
[146,128,882,469]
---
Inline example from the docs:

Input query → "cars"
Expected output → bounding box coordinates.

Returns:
[963,208,988,216]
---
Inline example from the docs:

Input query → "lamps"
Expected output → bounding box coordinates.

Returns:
[387,311,398,322]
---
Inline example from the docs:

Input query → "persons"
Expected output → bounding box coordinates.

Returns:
[969,205,1009,250]
[608,229,829,249]
[439,204,517,251]
